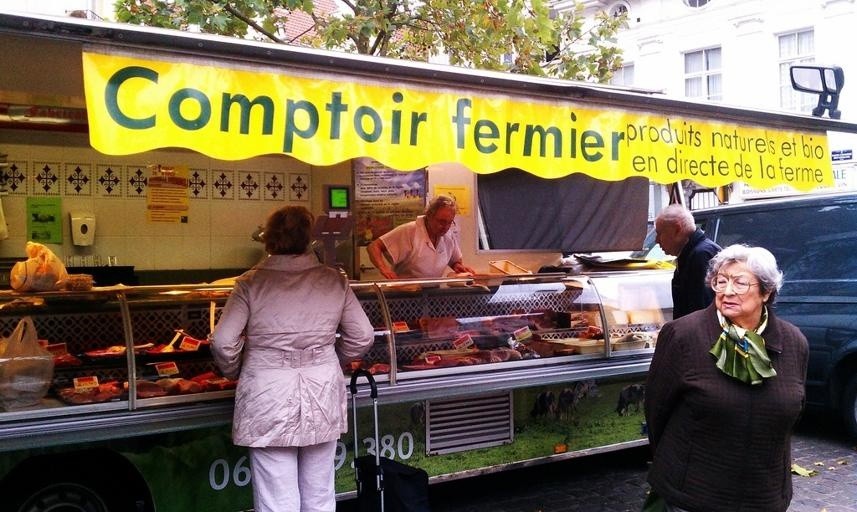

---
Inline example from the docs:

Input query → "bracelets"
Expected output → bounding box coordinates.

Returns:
[374,263,385,268]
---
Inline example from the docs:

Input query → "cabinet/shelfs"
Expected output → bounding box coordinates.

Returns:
[1,267,676,424]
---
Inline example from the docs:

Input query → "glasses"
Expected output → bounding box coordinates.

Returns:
[431,215,458,228]
[711,274,763,296]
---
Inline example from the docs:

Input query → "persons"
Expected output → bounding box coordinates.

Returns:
[208,205,374,511]
[366,194,475,287]
[643,244,809,511]
[656,203,726,320]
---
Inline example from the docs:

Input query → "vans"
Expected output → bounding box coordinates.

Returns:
[629,193,856,443]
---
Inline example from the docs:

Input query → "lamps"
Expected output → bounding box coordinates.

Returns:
[789,63,843,120]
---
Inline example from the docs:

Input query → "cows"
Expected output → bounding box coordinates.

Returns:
[528,391,555,418]
[553,388,576,421]
[613,384,648,417]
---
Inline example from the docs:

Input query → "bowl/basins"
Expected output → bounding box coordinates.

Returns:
[63,273,94,291]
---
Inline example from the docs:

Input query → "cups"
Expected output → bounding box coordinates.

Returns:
[66,253,119,266]
